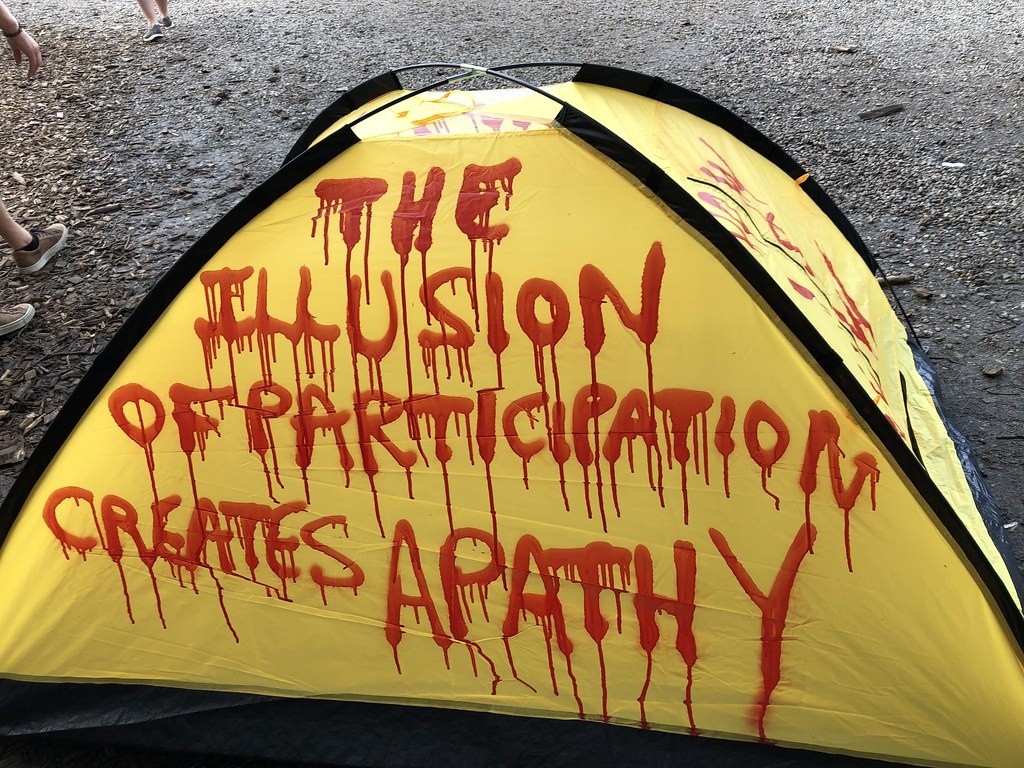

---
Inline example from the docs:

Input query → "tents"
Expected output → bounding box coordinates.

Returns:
[0,58,1024,768]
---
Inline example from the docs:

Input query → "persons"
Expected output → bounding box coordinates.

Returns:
[135,1,175,40]
[0,2,70,336]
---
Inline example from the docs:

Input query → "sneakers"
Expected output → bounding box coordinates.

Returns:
[142,24,164,41]
[0,303,36,337]
[157,16,176,30]
[13,223,67,276]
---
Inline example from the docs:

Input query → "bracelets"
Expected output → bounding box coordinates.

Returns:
[2,22,21,37]
